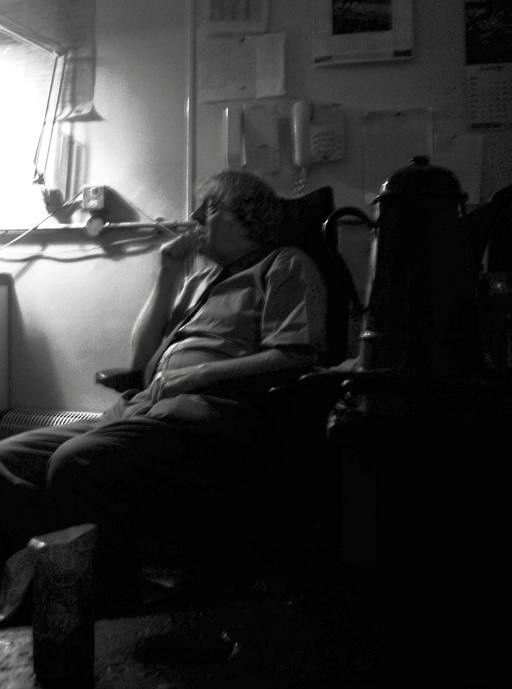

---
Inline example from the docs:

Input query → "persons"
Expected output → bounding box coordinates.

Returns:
[0,168,332,629]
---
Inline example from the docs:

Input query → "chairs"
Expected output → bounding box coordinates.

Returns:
[95,187,349,623]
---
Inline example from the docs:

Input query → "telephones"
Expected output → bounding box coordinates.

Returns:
[223,101,283,171]
[292,99,348,167]
